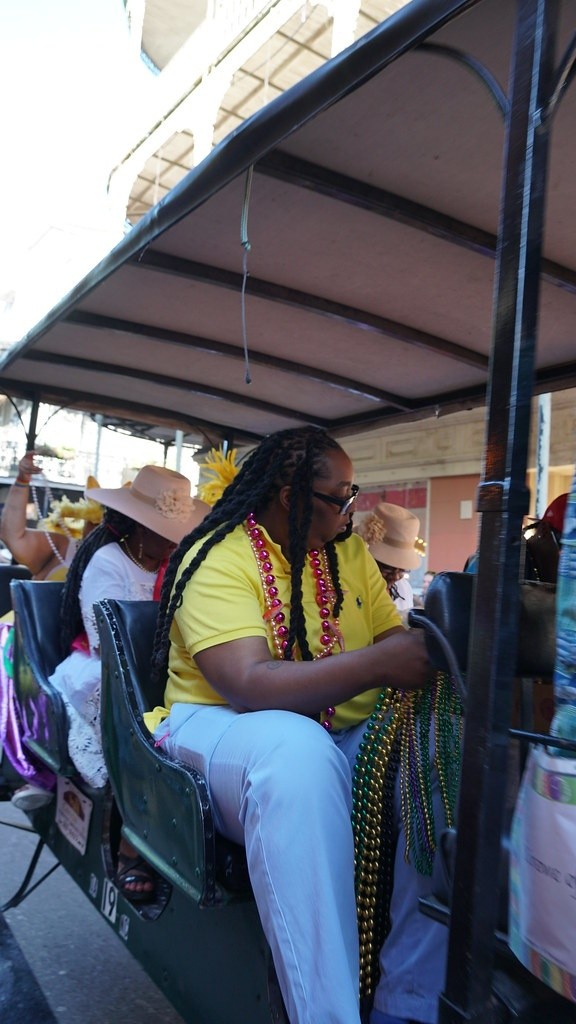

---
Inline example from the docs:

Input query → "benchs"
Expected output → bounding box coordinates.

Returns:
[0,563,563,908]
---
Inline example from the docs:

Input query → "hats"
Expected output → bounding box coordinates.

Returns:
[352,502,423,571]
[539,493,569,531]
[84,464,213,545]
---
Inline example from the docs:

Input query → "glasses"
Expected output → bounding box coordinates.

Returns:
[302,484,360,516]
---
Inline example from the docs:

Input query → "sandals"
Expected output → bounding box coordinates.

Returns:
[10,784,55,811]
[114,846,158,901]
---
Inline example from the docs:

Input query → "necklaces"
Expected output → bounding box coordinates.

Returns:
[31,471,77,569]
[351,669,466,998]
[124,539,162,574]
[242,513,346,733]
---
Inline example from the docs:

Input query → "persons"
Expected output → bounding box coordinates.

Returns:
[141,425,466,1024]
[464,492,568,585]
[351,503,423,630]
[422,571,435,601]
[0,450,215,902]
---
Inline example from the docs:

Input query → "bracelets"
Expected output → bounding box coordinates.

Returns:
[15,478,29,487]
